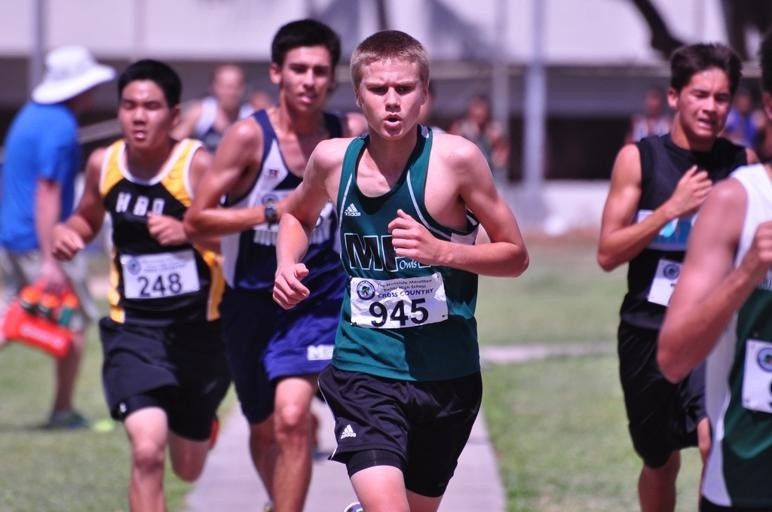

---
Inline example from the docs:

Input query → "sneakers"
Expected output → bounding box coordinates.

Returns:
[48,411,91,429]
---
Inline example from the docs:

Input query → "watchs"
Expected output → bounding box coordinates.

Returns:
[265,198,281,225]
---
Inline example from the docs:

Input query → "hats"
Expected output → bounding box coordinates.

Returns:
[30,44,116,104]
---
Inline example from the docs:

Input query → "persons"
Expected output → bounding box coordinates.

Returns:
[722,85,768,167]
[654,33,769,512]
[51,60,231,511]
[181,18,373,512]
[625,88,673,142]
[248,87,273,111]
[597,43,760,511]
[168,63,259,151]
[0,47,118,427]
[450,93,513,243]
[265,29,530,512]
